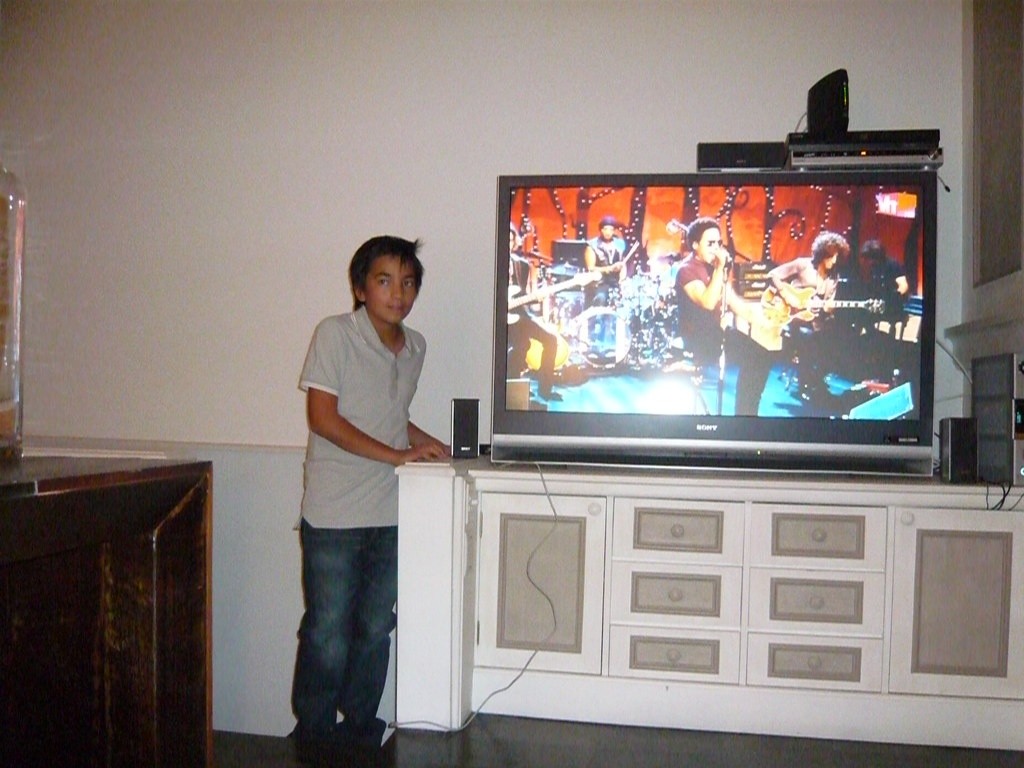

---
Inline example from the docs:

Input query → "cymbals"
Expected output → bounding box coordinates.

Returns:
[515,250,553,262]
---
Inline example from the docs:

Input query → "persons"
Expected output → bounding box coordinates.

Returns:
[507,225,563,412]
[768,232,909,416]
[675,218,753,415]
[291,236,451,768]
[585,217,628,355]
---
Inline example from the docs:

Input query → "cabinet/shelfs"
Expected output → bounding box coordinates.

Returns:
[386,458,1024,752]
[0,455,218,768]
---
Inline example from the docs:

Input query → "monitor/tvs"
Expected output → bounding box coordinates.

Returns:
[487,169,939,475]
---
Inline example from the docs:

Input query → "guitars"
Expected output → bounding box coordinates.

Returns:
[509,270,605,326]
[762,282,886,327]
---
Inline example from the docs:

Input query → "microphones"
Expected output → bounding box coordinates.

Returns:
[725,255,733,263]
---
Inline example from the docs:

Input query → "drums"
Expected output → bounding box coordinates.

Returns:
[569,305,633,370]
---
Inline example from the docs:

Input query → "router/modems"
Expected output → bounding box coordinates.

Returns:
[806,69,848,134]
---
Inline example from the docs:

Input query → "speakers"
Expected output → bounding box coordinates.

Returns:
[450,398,480,458]
[939,417,980,486]
